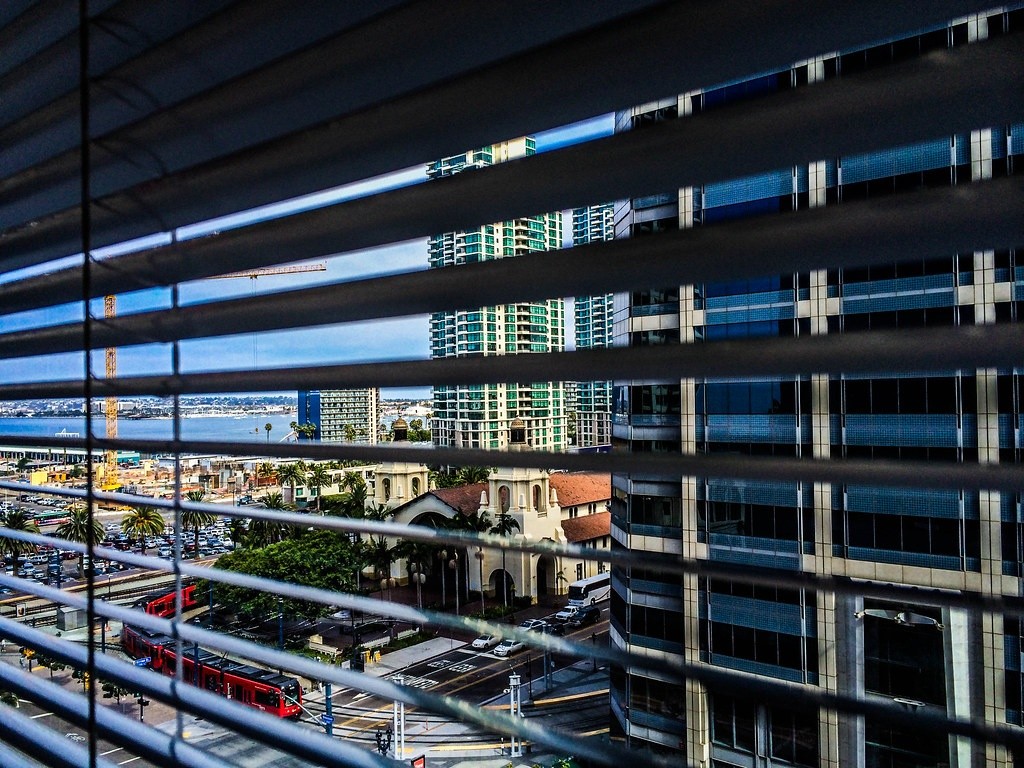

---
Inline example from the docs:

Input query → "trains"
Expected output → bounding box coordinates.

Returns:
[121,580,302,724]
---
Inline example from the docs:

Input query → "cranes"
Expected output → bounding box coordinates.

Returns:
[97,258,327,510]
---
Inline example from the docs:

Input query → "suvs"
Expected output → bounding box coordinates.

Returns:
[566,606,602,628]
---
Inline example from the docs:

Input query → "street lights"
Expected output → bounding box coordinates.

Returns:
[590,631,599,672]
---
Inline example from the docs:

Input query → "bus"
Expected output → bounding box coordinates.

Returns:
[566,570,612,609]
[34,510,75,526]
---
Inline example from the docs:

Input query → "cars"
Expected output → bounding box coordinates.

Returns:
[472,617,566,658]
[0,517,236,592]
[1,489,70,522]
[556,604,577,622]
